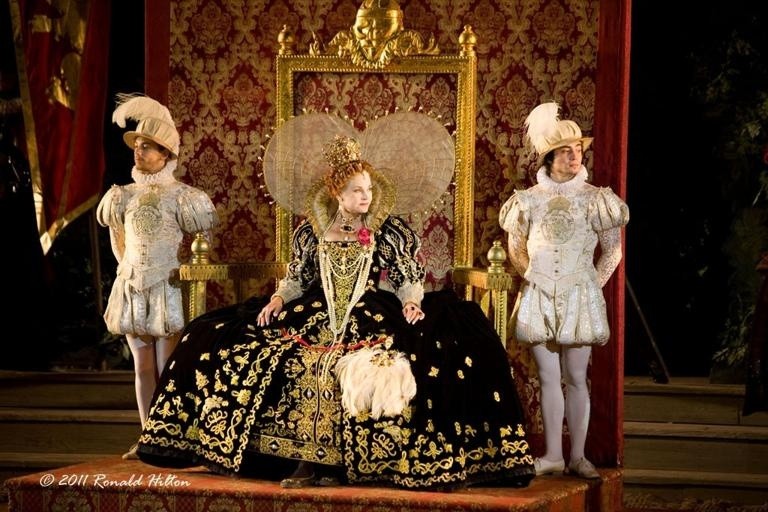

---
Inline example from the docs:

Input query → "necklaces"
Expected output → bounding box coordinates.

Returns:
[336,209,362,235]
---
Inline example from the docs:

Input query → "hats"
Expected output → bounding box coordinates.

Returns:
[111,94,181,159]
[524,103,594,167]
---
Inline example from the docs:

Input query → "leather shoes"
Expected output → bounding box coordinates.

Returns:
[317,476,340,487]
[281,469,316,488]
[532,456,566,478]
[567,456,600,478]
[122,444,140,462]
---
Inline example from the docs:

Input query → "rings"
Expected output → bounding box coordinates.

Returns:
[410,306,415,311]
[419,311,423,317]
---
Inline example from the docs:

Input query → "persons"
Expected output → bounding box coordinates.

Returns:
[496,99,633,483]
[99,95,220,460]
[255,135,430,492]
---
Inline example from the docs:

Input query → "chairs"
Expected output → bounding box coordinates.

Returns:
[177,0,514,351]
